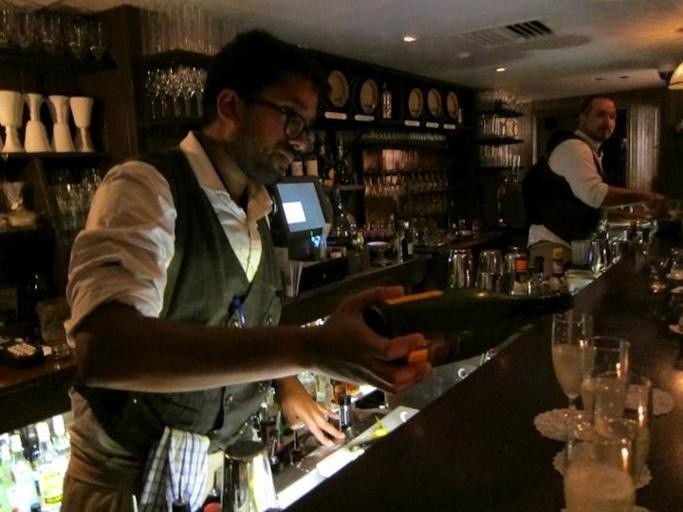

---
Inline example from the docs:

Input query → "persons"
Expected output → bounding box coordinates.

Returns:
[58,26,434,512]
[527,89,678,271]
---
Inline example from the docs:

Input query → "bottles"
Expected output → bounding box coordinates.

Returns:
[382,80,394,119]
[354,213,447,263]
[360,286,575,370]
[289,128,357,184]
[330,186,353,250]
[251,372,355,440]
[448,213,479,239]
[0,414,72,511]
[504,244,567,297]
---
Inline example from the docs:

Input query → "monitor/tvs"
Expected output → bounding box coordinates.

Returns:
[264,175,329,244]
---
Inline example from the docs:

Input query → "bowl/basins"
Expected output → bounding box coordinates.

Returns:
[642,198,682,222]
[329,71,378,113]
[408,89,458,120]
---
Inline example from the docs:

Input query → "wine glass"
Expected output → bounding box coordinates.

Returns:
[360,127,448,145]
[0,0,108,59]
[552,308,654,511]
[364,167,450,216]
[143,64,206,118]
[476,87,523,167]
[0,89,97,154]
[51,166,105,218]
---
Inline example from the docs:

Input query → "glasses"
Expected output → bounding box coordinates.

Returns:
[241,93,321,145]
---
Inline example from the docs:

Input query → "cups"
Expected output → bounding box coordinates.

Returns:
[570,240,601,270]
[447,247,502,294]
[281,260,306,297]
[39,300,72,357]
[221,442,279,512]
